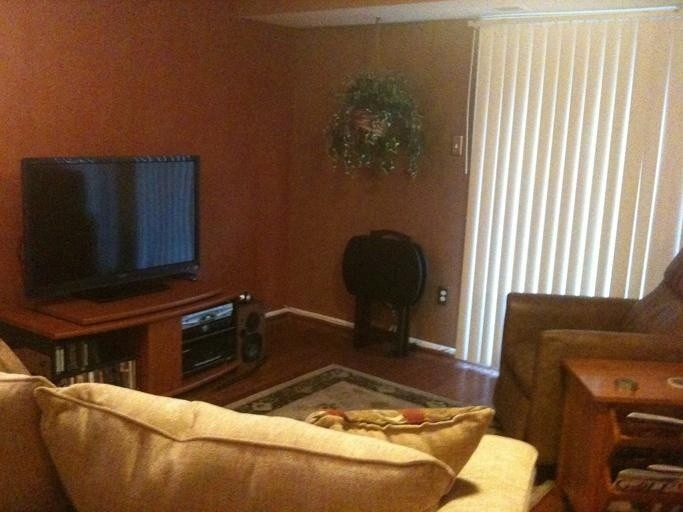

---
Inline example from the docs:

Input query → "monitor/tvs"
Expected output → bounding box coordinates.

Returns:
[20,155,201,306]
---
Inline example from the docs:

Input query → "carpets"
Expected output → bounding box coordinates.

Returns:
[215,362,468,420]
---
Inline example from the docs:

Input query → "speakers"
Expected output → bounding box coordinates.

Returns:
[14,348,53,385]
[236,299,265,378]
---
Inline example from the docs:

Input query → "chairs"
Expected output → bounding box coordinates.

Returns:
[493,248,683,466]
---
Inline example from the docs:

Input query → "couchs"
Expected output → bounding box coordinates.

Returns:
[1,339,541,511]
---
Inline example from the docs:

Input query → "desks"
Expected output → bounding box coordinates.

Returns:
[555,355,683,512]
[0,271,266,396]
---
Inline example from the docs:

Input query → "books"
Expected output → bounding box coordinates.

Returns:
[26,340,92,376]
[56,359,137,391]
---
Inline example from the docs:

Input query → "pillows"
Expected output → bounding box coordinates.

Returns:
[32,382,455,511]
[306,405,496,477]
[0,373,71,511]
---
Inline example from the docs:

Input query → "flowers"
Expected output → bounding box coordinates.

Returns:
[326,73,430,181]
